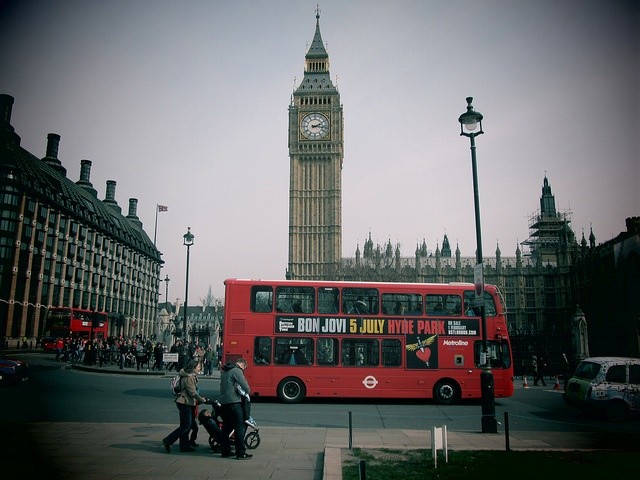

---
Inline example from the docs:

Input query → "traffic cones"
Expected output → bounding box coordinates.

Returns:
[554,374,561,390]
[523,375,529,389]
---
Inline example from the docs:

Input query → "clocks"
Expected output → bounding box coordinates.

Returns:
[298,112,331,139]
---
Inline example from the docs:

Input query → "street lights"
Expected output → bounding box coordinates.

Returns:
[182,226,194,346]
[458,98,498,433]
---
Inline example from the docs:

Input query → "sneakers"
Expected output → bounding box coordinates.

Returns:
[180,446,196,452]
[543,384,548,386]
[152,367,172,371]
[204,374,206,377]
[236,453,253,460]
[147,368,149,371]
[121,367,125,369]
[210,374,212,377]
[534,384,539,385]
[190,440,200,447]
[221,452,235,457]
[163,438,171,452]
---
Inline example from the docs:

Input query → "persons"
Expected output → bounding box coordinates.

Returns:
[531,355,537,386]
[276,343,292,364]
[191,384,199,447]
[394,301,406,315]
[356,295,371,314]
[55,333,217,374]
[219,357,254,459]
[534,356,547,386]
[162,359,197,452]
[293,299,304,314]
[201,408,235,439]
[294,344,308,364]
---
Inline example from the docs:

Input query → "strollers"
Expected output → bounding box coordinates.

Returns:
[197,399,260,453]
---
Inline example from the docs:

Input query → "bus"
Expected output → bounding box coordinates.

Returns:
[43,308,108,351]
[218,278,513,403]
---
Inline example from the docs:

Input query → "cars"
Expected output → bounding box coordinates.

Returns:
[563,357,639,422]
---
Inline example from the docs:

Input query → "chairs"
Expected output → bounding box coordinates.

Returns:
[268,299,469,316]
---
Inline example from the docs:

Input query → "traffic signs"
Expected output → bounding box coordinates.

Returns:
[163,353,180,363]
[472,264,486,305]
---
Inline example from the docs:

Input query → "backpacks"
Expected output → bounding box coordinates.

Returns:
[170,374,186,396]
